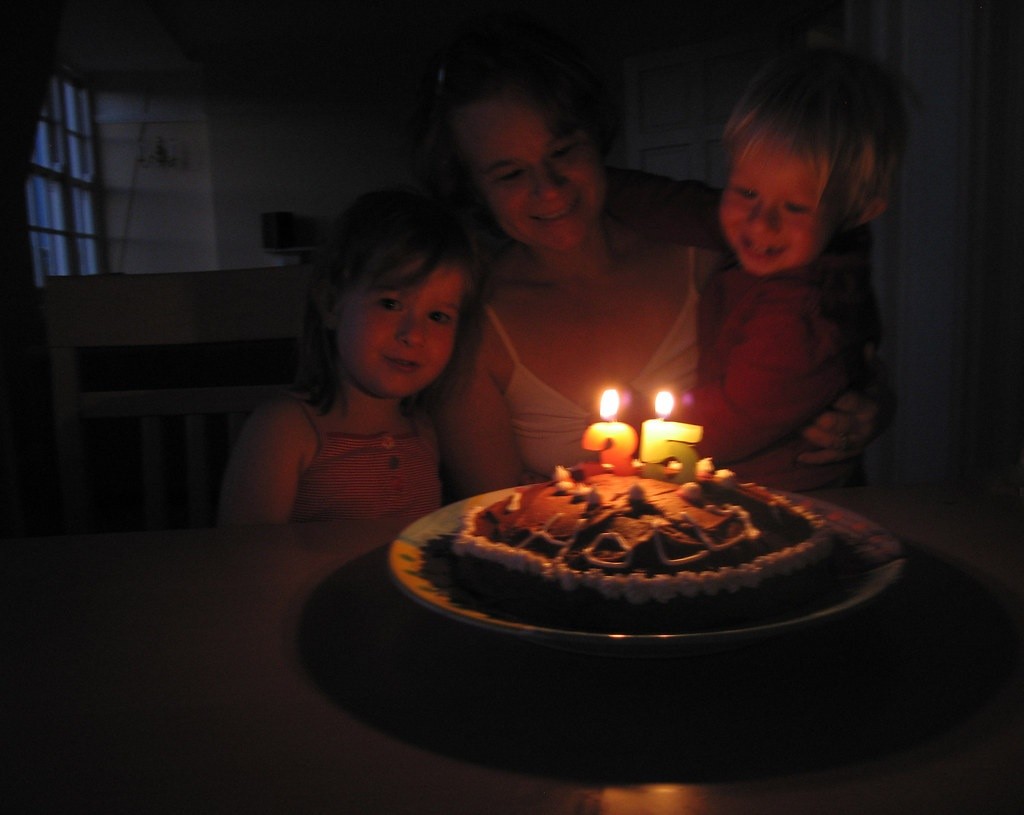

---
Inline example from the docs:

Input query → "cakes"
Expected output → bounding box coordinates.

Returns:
[444,460,846,629]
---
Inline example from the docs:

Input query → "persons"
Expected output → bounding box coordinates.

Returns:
[463,51,905,491]
[217,188,481,529]
[407,10,896,496]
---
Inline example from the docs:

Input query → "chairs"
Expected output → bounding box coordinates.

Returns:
[44,267,310,533]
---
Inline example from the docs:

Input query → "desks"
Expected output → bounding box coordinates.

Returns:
[0,482,1024,815]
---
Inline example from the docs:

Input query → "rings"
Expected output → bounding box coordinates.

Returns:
[833,432,848,452]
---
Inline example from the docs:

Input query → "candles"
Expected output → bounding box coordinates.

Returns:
[577,389,704,486]
